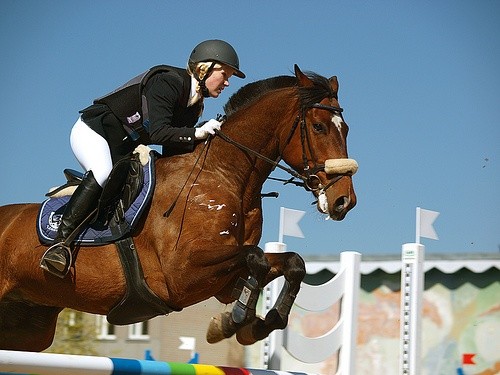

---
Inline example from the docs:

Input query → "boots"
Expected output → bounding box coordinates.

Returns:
[41,170,105,276]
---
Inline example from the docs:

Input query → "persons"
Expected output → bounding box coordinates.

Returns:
[43,39,246,272]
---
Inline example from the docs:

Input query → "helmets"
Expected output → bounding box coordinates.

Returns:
[188,39,246,79]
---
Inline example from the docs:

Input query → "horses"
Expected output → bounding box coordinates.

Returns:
[0,63,357,352]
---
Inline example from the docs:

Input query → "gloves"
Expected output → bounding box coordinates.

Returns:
[195,118,224,139]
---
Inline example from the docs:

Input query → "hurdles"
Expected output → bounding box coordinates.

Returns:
[0,206,440,374]
